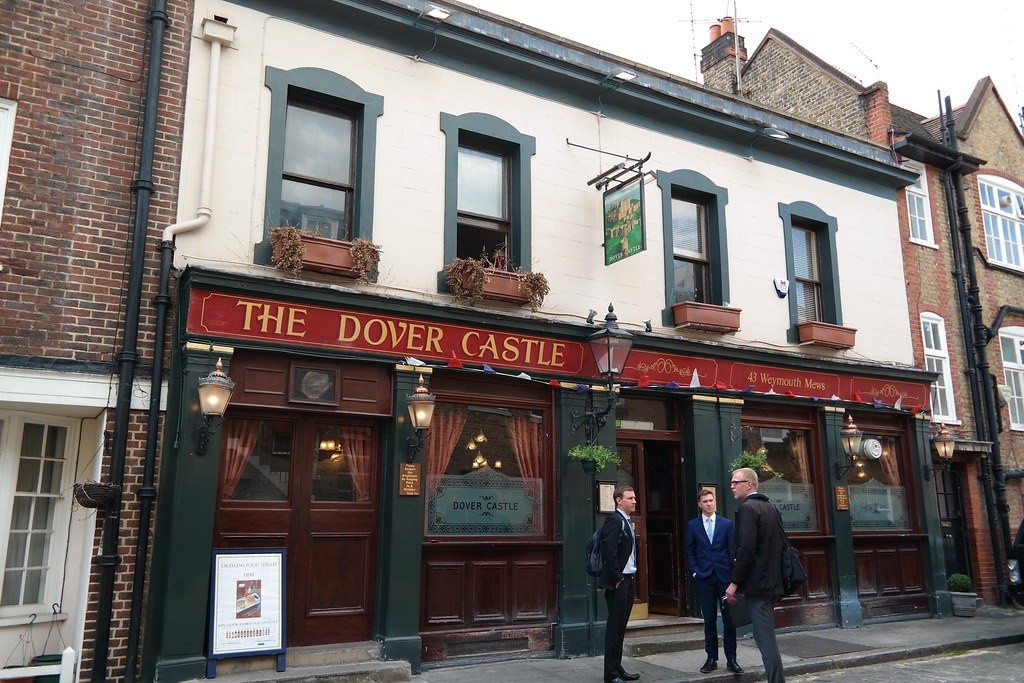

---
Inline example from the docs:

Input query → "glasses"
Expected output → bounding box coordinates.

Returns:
[730,481,749,486]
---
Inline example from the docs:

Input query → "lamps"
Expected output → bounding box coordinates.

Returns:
[406,374,436,464]
[318,429,343,452]
[754,128,789,139]
[474,415,487,443]
[471,445,502,469]
[836,414,864,480]
[195,358,236,456]
[466,426,478,449]
[600,68,639,89]
[415,4,452,30]
[586,308,598,325]
[643,319,652,332]
[571,303,635,434]
[923,422,956,482]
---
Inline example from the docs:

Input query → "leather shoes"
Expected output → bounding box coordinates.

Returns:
[700,657,718,673]
[618,671,640,682]
[726,659,743,673]
[608,677,626,683]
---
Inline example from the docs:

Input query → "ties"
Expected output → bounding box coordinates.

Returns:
[706,518,713,545]
[628,519,637,565]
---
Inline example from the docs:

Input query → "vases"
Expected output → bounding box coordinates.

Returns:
[73,484,118,509]
[672,301,742,333]
[797,321,858,349]
[32,654,61,683]
[0,666,33,683]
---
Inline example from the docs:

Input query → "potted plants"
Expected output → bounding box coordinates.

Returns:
[443,251,550,314]
[948,574,978,617]
[727,449,784,480]
[568,444,622,476]
[270,228,380,286]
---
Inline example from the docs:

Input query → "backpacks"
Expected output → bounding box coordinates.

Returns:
[585,514,624,577]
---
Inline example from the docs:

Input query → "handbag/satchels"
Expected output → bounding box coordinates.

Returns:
[782,546,807,595]
[730,593,753,630]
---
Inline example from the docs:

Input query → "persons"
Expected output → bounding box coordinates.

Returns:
[685,489,744,673]
[599,486,640,683]
[726,467,791,683]
